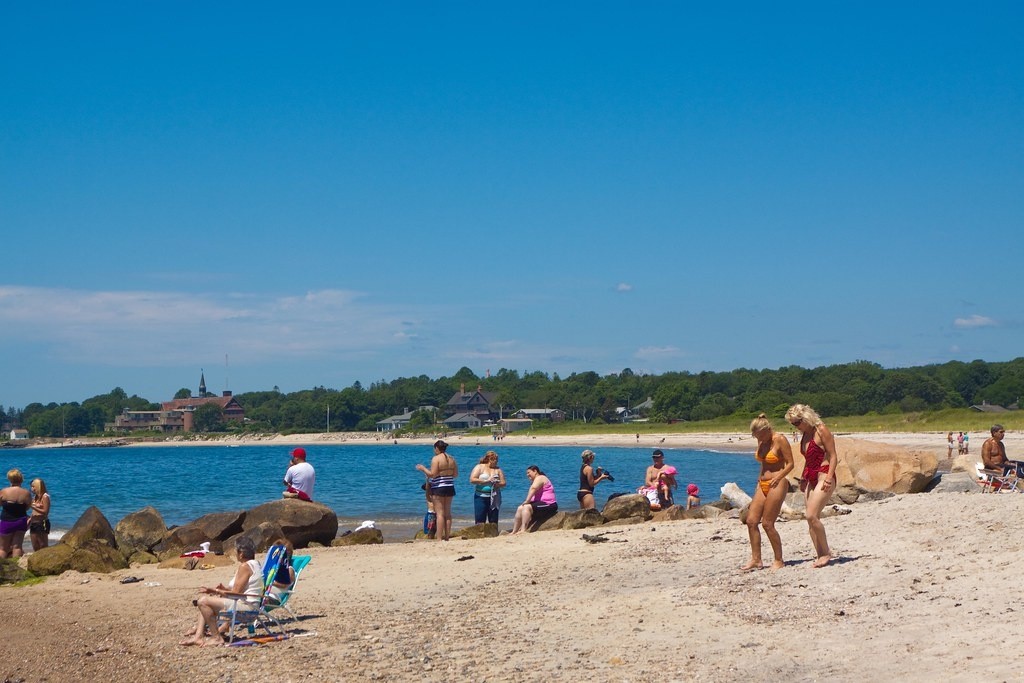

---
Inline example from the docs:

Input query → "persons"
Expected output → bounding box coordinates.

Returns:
[656,466,677,500]
[0,469,32,560]
[741,413,794,570]
[283,447,315,500]
[184,538,295,635]
[27,477,50,552]
[981,423,1017,477]
[178,537,265,646]
[963,431,969,454]
[421,475,436,539]
[947,431,953,459]
[416,440,457,541]
[576,450,609,509]
[785,404,837,567]
[470,451,506,528]
[510,466,558,534]
[686,483,700,510]
[958,432,964,455]
[645,450,678,512]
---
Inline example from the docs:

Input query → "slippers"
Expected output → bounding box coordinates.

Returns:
[119,576,145,584]
[199,564,215,570]
[183,556,198,571]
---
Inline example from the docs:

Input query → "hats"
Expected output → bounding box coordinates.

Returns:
[290,448,306,458]
[664,466,677,475]
[687,483,699,496]
[652,449,664,457]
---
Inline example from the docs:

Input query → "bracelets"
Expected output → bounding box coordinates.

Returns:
[824,479,832,485]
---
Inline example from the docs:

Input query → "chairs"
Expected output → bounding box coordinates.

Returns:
[263,554,311,635]
[978,461,1024,494]
[212,545,289,646]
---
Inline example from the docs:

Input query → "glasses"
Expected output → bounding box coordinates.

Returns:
[791,418,803,427]
